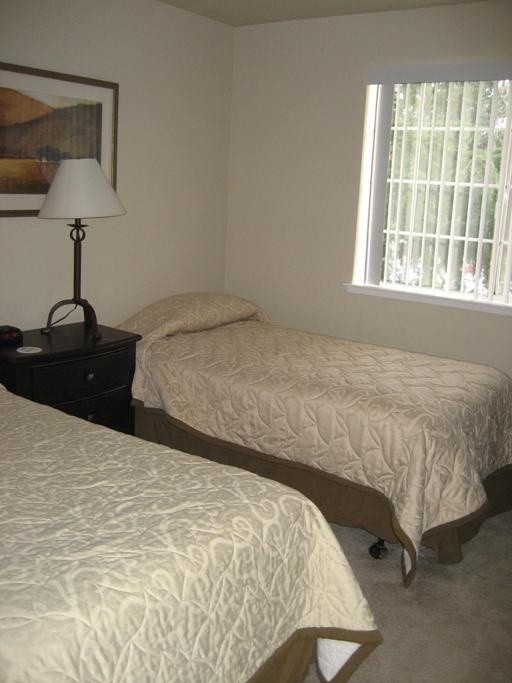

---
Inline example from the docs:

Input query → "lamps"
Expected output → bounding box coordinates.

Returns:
[38,157,125,339]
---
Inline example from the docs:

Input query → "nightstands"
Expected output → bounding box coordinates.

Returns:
[2,322,146,442]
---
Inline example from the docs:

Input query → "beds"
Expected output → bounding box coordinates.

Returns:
[110,293,510,588]
[0,385,386,682]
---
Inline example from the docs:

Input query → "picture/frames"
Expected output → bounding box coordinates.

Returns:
[0,60,119,217]
